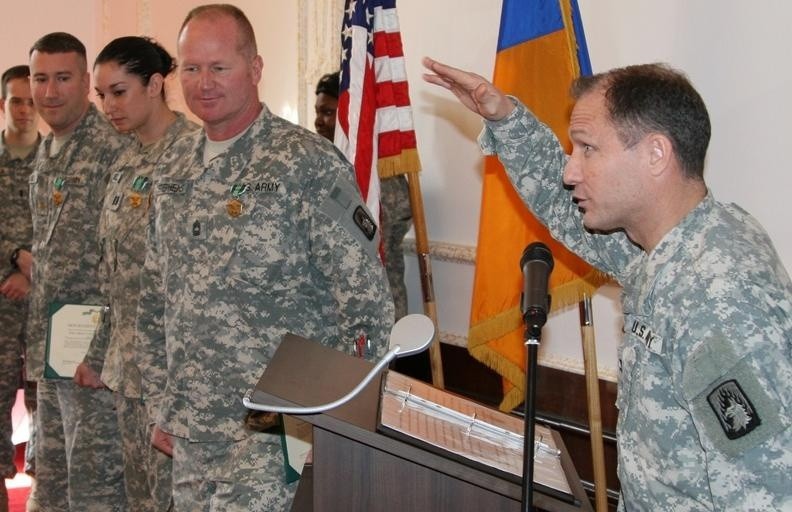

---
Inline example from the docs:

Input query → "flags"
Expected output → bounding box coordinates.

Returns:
[468,0,612,415]
[335,0,421,266]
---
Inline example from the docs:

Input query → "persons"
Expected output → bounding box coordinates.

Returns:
[134,4,396,512]
[315,72,412,323]
[0,66,46,512]
[24,32,138,512]
[421,57,792,512]
[93,37,199,512]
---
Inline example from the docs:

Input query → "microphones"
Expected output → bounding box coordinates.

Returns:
[243,314,435,415]
[520,242,554,338]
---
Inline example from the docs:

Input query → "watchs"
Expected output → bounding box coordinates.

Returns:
[11,248,20,264]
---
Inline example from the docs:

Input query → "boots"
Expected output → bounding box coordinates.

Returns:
[25,477,38,512]
